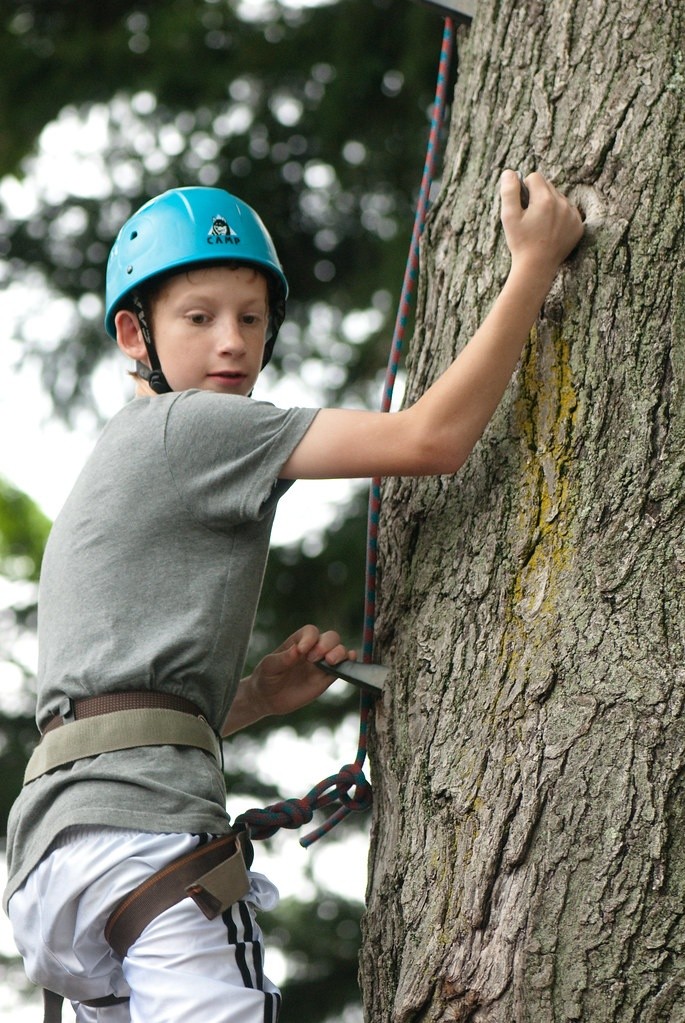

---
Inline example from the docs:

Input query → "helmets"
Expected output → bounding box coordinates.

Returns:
[102,184,290,339]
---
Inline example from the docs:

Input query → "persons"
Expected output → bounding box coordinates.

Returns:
[4,165,584,1022]
